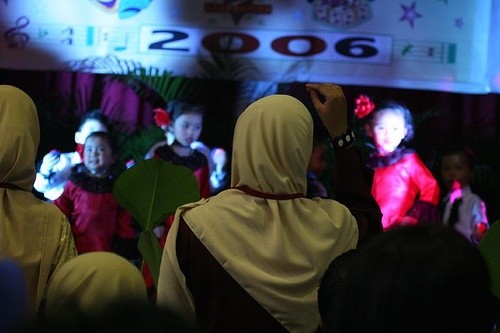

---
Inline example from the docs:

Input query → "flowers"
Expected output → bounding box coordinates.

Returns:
[153,108,172,131]
[354,94,376,119]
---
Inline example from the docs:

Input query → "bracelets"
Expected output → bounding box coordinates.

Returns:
[328,126,356,151]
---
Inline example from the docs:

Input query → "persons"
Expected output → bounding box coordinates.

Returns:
[0,82,499,333]
